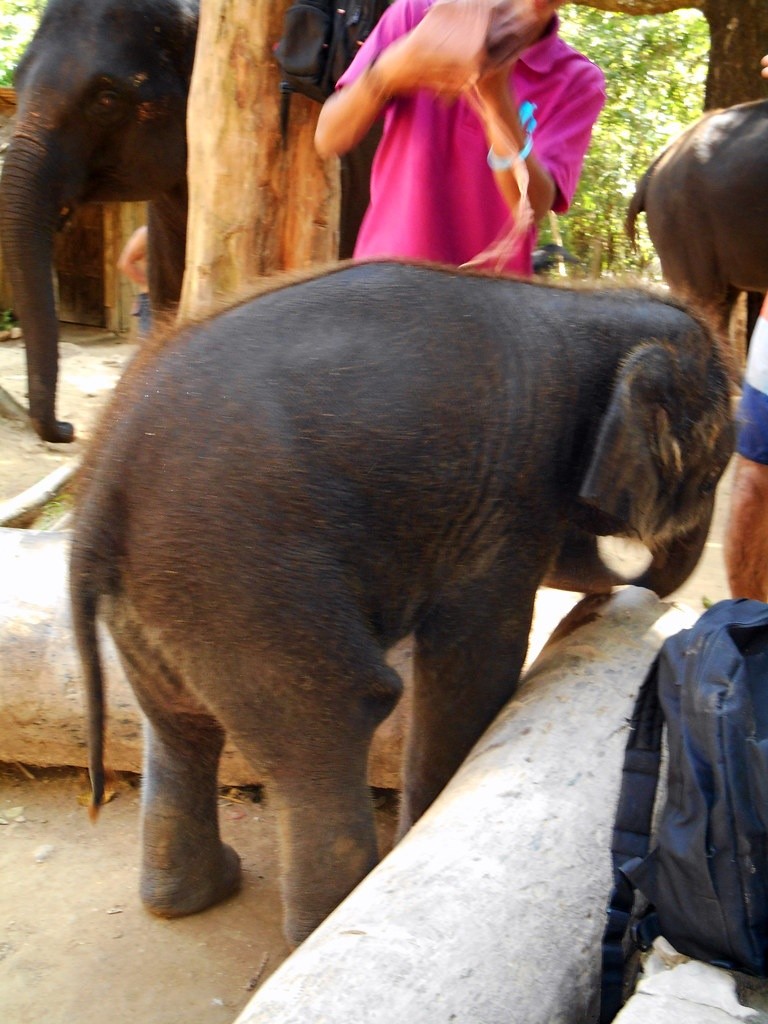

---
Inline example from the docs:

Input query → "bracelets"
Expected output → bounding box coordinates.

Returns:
[485,132,533,170]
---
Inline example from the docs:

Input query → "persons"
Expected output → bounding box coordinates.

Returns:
[725,292,768,603]
[311,0,608,286]
[116,225,150,343]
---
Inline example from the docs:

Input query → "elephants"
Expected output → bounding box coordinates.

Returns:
[69,253,739,959]
[0,0,379,443]
[627,95,768,371]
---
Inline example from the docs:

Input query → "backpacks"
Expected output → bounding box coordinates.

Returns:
[590,598,768,1024]
